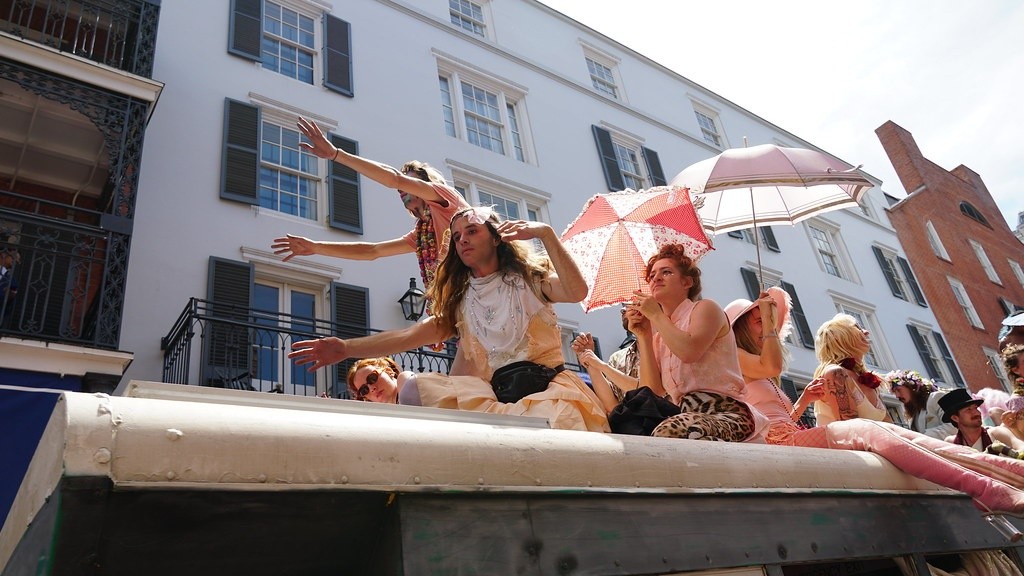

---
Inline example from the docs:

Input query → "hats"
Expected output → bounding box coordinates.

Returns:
[937,388,984,423]
[724,299,778,328]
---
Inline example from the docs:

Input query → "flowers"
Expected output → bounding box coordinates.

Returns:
[885,369,923,392]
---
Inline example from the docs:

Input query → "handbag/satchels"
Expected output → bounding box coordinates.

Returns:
[490,361,565,403]
[607,386,681,436]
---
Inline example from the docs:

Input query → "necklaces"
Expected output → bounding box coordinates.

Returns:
[767,378,806,430]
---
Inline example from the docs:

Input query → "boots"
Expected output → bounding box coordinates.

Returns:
[853,418,1024,543]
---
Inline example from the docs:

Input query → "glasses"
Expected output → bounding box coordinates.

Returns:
[356,365,390,398]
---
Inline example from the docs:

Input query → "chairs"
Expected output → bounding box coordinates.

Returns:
[214,365,283,394]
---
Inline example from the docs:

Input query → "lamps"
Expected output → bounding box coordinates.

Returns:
[397,277,427,322]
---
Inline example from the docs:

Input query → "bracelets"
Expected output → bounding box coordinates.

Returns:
[762,333,778,342]
[331,148,338,161]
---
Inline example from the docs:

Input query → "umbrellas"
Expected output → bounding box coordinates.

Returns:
[559,183,716,314]
[673,138,874,291]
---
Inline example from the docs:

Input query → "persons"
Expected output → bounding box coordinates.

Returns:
[271,116,482,377]
[885,370,957,441]
[287,207,611,434]
[625,243,771,443]
[349,356,414,405]
[975,310,1024,441]
[725,286,1024,542]
[814,315,893,426]
[572,308,673,415]
[937,388,1024,459]
